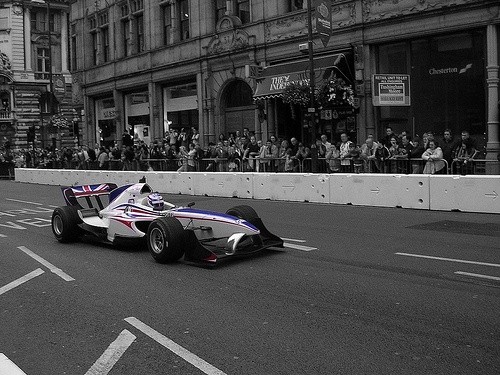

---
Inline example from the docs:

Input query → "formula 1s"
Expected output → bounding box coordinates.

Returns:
[50,176,284,270]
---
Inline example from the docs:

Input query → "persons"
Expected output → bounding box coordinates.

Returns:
[242,136,260,172]
[97,146,109,169]
[380,127,400,148]
[455,131,472,159]
[257,140,267,172]
[397,135,414,162]
[290,137,303,172]
[388,136,400,174]
[278,140,295,173]
[309,138,326,173]
[439,129,457,174]
[423,130,437,151]
[133,133,142,146]
[296,143,311,172]
[229,144,241,171]
[325,145,340,172]
[265,139,279,172]
[321,135,331,156]
[164,127,200,143]
[219,133,225,143]
[422,139,446,175]
[270,135,280,149]
[232,130,242,148]
[214,141,228,172]
[0,143,186,169]
[347,143,360,173]
[239,127,251,148]
[358,139,378,172]
[339,133,354,172]
[398,129,411,143]
[147,192,165,211]
[194,143,204,171]
[184,143,196,172]
[411,138,423,158]
[222,140,231,157]
[361,134,378,149]
[369,138,391,174]
[122,129,134,146]
[205,142,217,171]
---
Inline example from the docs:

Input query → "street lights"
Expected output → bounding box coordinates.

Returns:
[30,0,55,117]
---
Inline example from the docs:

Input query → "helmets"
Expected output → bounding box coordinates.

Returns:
[147,193,164,211]
